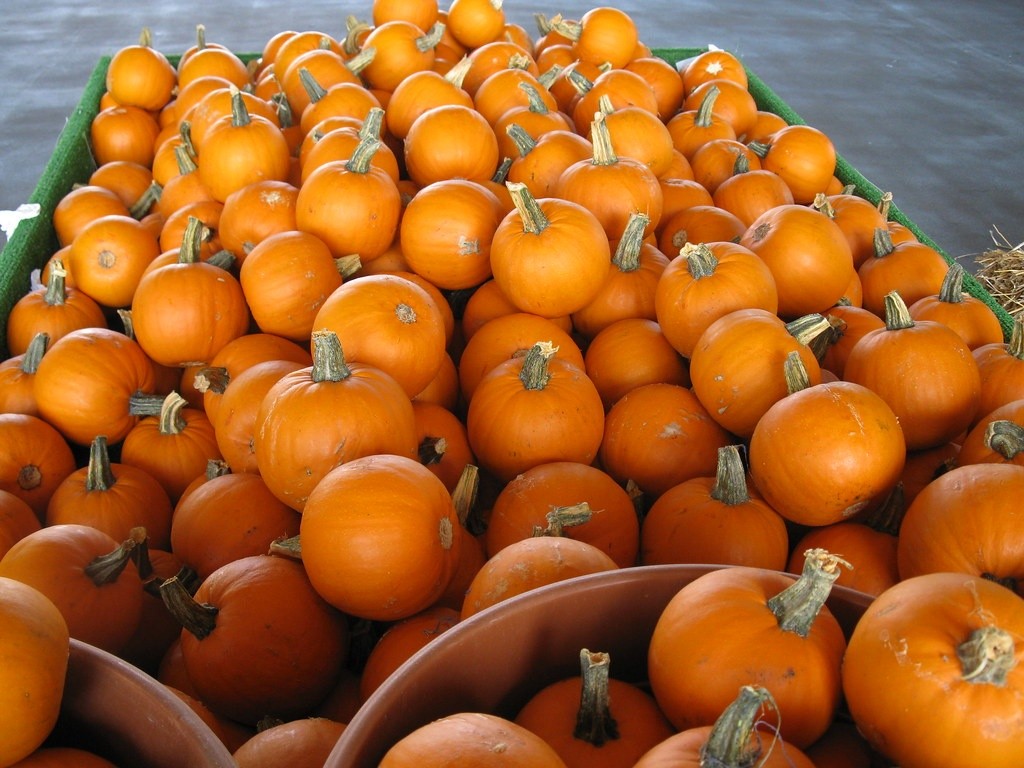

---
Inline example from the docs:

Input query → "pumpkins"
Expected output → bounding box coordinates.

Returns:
[0,0,1024,768]
